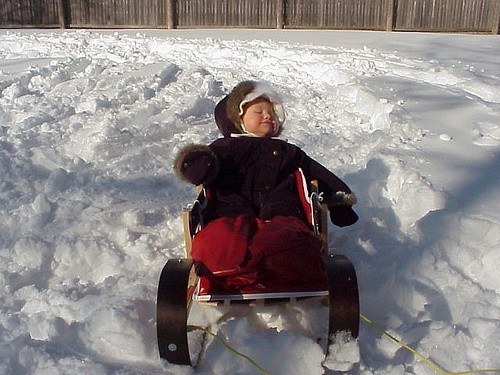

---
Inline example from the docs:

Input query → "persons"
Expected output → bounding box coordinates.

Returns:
[175,80,358,295]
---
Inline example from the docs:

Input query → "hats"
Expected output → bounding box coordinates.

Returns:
[238,89,285,133]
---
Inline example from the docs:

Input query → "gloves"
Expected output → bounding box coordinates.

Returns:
[179,151,217,187]
[328,206,359,227]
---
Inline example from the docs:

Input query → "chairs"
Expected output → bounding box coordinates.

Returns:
[156,180,360,372]
